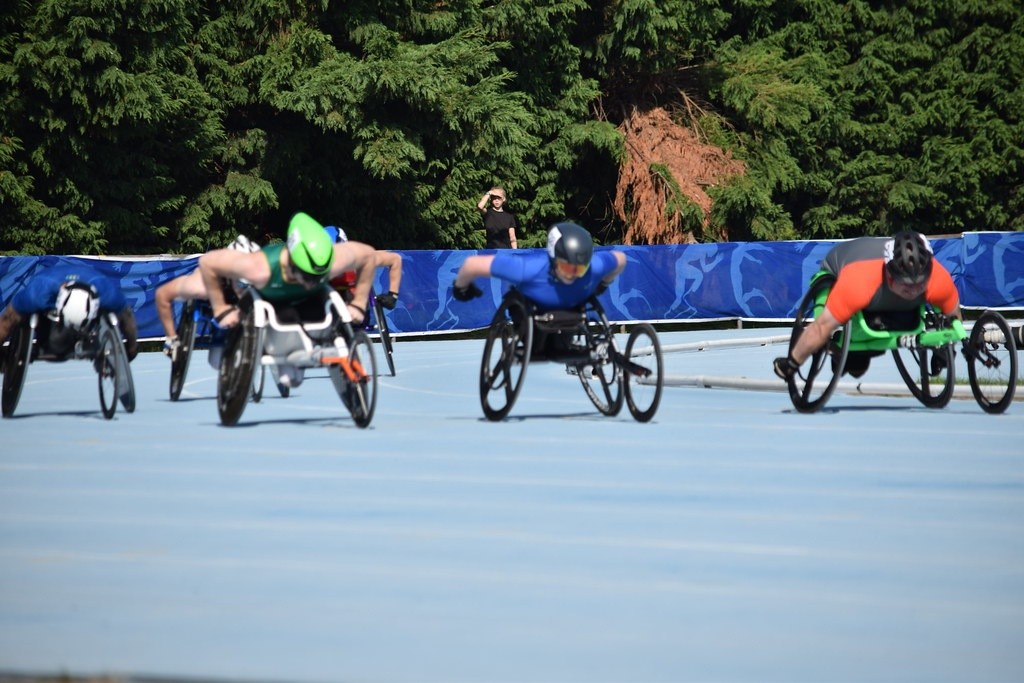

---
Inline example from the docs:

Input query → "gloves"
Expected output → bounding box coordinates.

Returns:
[164,337,184,363]
[374,291,398,311]
[452,280,481,303]
[595,280,615,297]
[774,353,800,381]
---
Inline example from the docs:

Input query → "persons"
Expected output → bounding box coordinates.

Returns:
[198,212,377,332]
[774,232,962,380]
[155,235,261,361]
[323,226,402,310]
[0,265,139,372]
[452,222,626,310]
[478,186,517,250]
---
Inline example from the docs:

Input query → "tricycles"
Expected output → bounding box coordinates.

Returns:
[170,293,396,428]
[0,306,136,419]
[480,289,664,423]
[787,270,1019,414]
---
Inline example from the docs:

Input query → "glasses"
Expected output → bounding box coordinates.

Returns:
[554,257,591,277]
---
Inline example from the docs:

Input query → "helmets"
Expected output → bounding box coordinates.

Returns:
[54,281,99,331]
[883,233,933,285]
[226,235,262,290]
[286,213,333,275]
[546,224,593,266]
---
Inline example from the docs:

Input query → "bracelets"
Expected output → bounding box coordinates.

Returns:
[485,192,490,195]
